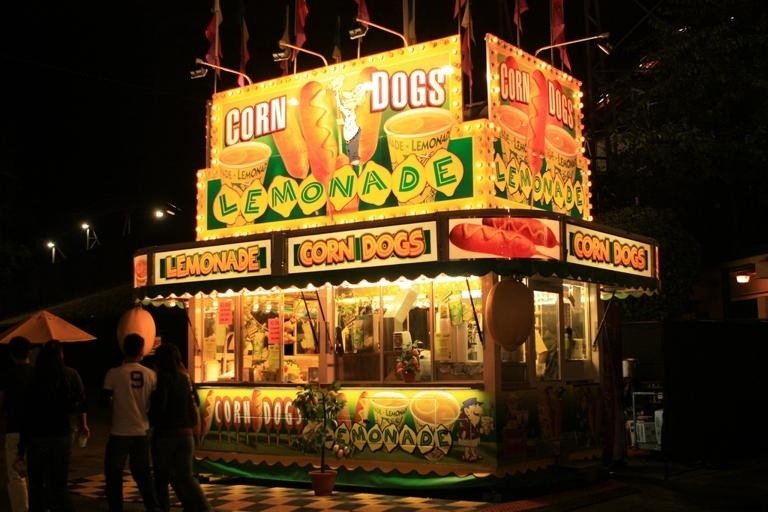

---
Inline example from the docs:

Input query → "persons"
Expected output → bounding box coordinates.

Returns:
[143,343,214,511]
[535,323,560,382]
[99,331,163,511]
[11,338,92,511]
[0,335,37,512]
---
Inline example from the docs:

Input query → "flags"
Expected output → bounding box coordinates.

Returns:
[406,0,415,37]
[356,0,369,22]
[277,32,287,69]
[295,0,309,48]
[240,17,250,63]
[205,15,223,76]
[454,0,465,18]
[550,0,571,70]
[332,20,341,58]
[514,0,528,32]
[461,34,472,75]
[461,4,476,44]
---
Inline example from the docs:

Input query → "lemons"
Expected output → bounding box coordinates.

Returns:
[485,277,534,353]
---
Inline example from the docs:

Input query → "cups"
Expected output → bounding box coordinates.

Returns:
[495,105,578,217]
[78,430,88,448]
[217,141,272,227]
[372,388,461,433]
[384,107,453,207]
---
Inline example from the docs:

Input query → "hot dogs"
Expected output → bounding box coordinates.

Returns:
[526,69,548,210]
[270,67,383,220]
[199,389,369,449]
[448,218,559,258]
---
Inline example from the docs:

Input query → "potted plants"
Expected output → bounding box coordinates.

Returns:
[292,379,348,495]
